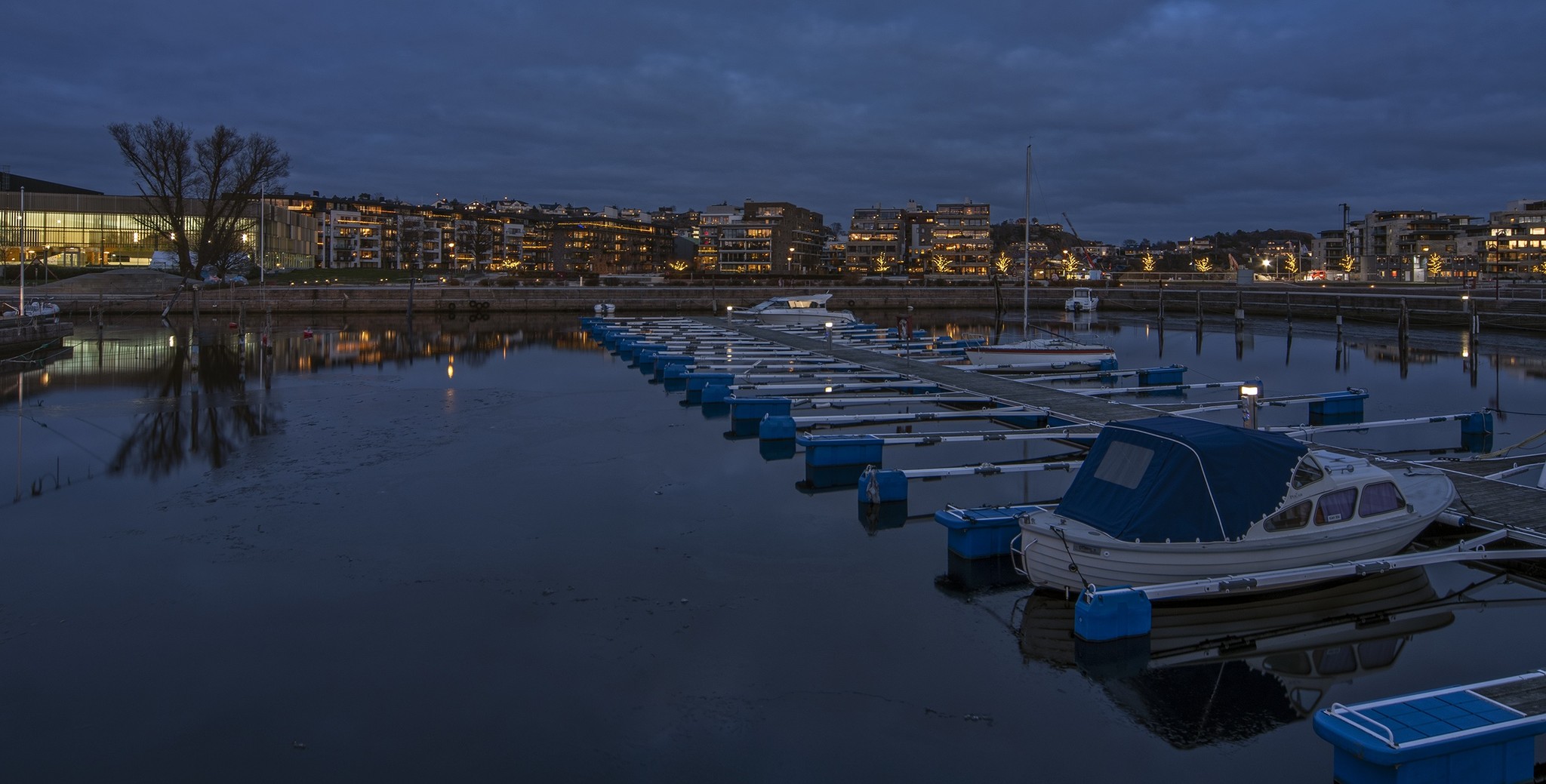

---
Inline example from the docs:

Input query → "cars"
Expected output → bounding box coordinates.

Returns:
[203,275,250,285]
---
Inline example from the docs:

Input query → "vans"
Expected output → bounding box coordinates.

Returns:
[1254,274,1272,281]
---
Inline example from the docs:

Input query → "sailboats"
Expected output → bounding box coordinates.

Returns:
[964,144,1120,373]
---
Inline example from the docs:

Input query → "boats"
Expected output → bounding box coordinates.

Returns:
[1011,411,1460,596]
[3,299,60,318]
[1312,668,1546,784]
[1065,287,1099,312]
[594,302,615,314]
[728,293,856,325]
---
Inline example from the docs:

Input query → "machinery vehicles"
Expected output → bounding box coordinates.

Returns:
[1060,212,1111,278]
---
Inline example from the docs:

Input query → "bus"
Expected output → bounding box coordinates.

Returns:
[1307,270,1326,279]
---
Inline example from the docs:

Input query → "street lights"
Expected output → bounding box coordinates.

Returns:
[1489,230,1506,302]
[1062,248,1068,279]
[449,238,455,285]
[1263,259,1270,274]
[788,247,795,273]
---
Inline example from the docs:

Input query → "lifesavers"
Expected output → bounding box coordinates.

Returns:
[469,301,490,310]
[848,300,855,307]
[469,313,489,322]
[447,313,456,319]
[449,303,456,310]
